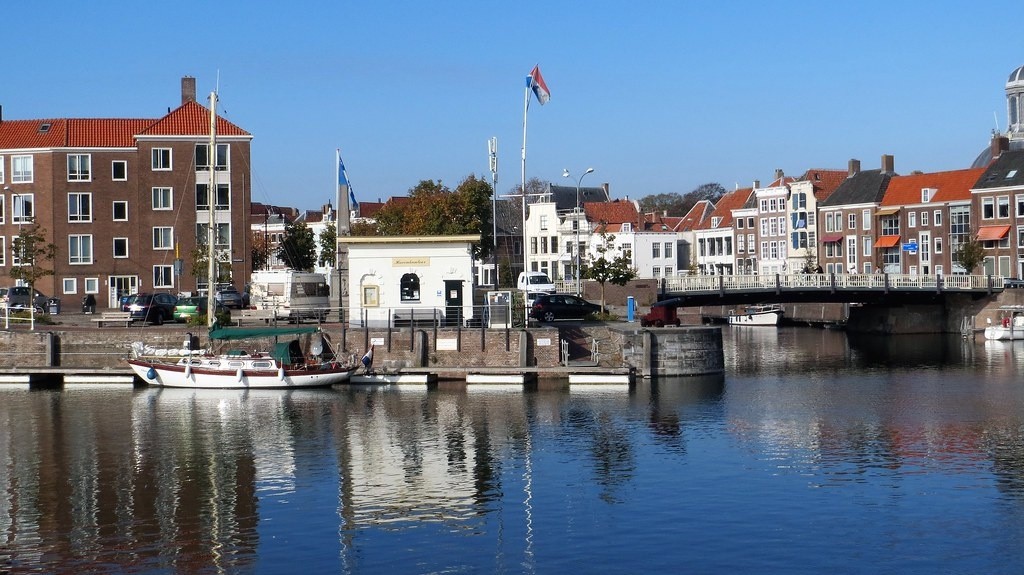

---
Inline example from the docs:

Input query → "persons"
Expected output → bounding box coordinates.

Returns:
[1002,312,1024,328]
[305,352,333,371]
[850,264,858,286]
[800,263,810,281]
[813,264,824,286]
[874,266,882,286]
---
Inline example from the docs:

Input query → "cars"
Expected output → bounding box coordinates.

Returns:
[202,285,243,308]
[522,291,551,313]
[174,296,233,323]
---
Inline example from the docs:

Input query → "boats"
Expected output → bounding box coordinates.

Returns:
[984,315,1024,343]
[728,304,786,326]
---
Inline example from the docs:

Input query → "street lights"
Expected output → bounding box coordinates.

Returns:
[263,206,283,270]
[563,167,595,297]
[4,185,23,286]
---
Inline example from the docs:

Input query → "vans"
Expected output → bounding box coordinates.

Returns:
[518,271,556,295]
[248,270,331,324]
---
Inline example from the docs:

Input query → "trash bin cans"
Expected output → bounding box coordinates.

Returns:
[82,295,96,315]
[49,298,60,315]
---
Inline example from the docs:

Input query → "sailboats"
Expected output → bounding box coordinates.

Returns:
[126,90,362,389]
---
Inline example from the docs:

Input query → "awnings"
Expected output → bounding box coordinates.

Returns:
[973,225,1010,239]
[818,236,842,242]
[873,235,901,246]
[874,210,899,215]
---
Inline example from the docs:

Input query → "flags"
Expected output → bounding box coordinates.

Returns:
[361,345,374,370]
[339,157,358,209]
[526,66,550,106]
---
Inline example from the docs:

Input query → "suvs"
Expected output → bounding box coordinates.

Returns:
[639,305,680,328]
[529,294,609,323]
[0,286,51,314]
[124,291,178,325]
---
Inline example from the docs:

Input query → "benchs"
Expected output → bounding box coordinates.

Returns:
[392,309,441,328]
[91,312,133,328]
[230,310,276,327]
